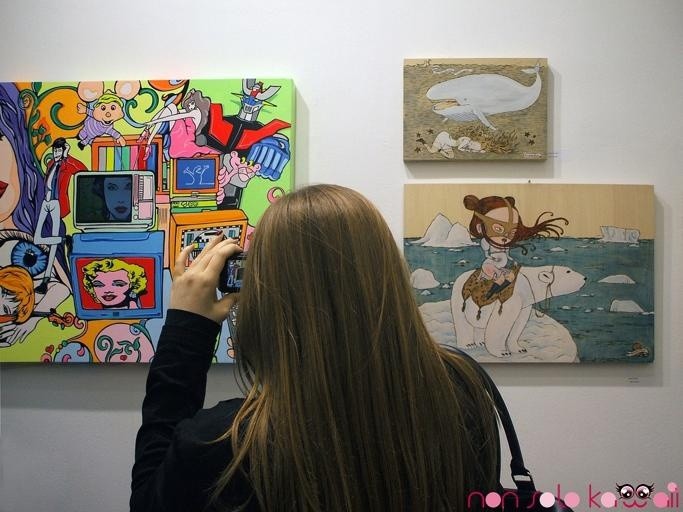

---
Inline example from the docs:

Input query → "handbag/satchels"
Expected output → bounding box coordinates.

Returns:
[496,473,572,512]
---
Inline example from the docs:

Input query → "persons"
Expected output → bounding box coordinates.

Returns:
[0,264,34,347]
[135,88,207,160]
[416,131,485,158]
[80,260,147,307]
[461,190,520,303]
[33,137,87,292]
[126,180,505,508]
[0,81,70,348]
[102,177,130,221]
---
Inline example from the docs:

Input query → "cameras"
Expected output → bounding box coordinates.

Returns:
[219,252,249,293]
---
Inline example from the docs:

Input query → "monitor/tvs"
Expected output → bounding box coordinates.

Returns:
[169,209,247,280]
[68,253,165,320]
[74,170,156,234]
